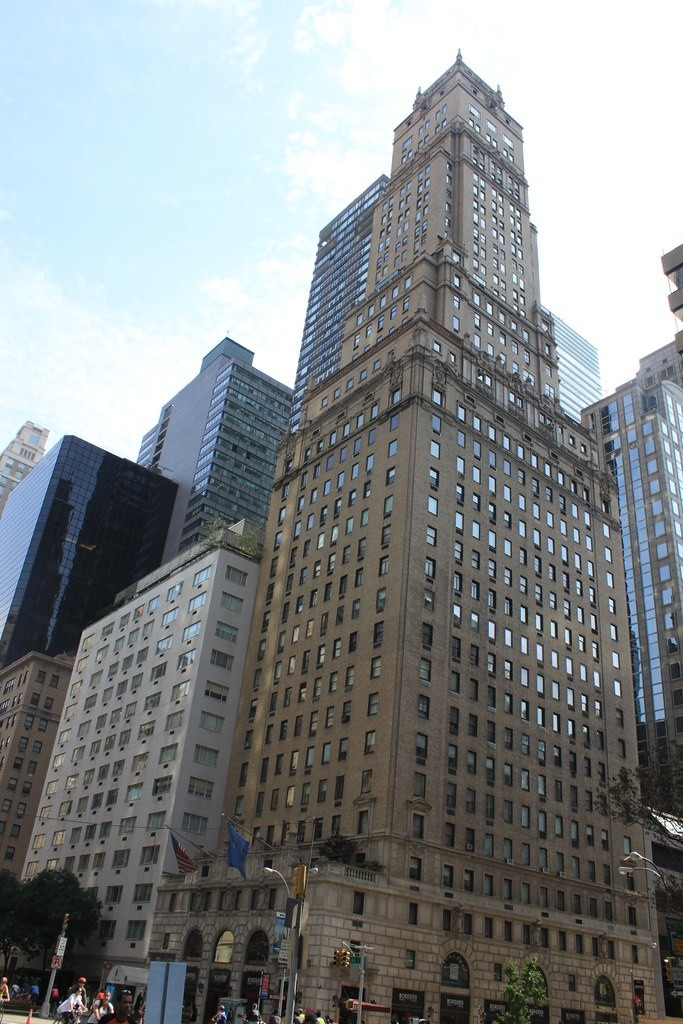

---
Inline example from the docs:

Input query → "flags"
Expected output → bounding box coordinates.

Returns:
[170,831,198,874]
[227,821,249,881]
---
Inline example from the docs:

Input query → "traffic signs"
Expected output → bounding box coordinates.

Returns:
[670,989,683,997]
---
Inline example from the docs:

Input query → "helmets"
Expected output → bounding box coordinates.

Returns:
[1,977,7,982]
[219,1005,224,1009]
[79,977,86,983]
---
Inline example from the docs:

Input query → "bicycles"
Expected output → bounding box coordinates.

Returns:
[51,1000,58,1017]
[0,997,8,1022]
[30,996,37,1012]
[23,992,28,1002]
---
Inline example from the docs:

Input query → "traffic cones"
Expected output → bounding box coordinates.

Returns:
[26,1009,33,1024]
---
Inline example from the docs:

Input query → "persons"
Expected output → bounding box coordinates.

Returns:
[212,1005,225,1024]
[0,974,146,1024]
[244,1003,431,1024]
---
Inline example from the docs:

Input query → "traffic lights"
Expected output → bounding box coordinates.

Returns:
[340,949,350,967]
[293,865,306,895]
[664,957,676,983]
[333,949,340,968]
[347,998,354,1010]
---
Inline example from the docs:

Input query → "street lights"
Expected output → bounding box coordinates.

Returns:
[263,868,291,1015]
[618,851,672,917]
